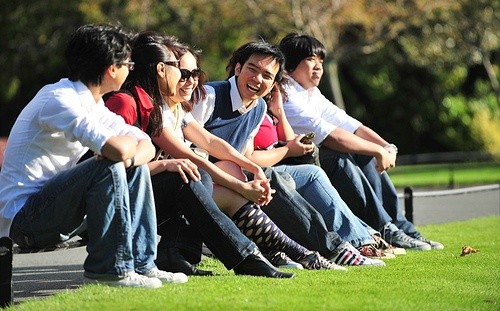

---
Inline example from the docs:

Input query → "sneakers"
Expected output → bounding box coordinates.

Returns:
[84,270,162,288]
[142,266,188,283]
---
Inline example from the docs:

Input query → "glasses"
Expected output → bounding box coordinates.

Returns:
[180,68,200,82]
[115,59,135,70]
[164,61,180,70]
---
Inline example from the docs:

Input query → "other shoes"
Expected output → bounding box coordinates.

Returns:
[233,252,297,279]
[263,250,304,270]
[416,234,444,250]
[298,251,347,270]
[326,240,386,266]
[157,260,215,276]
[354,243,396,259]
[391,229,431,251]
[373,236,407,255]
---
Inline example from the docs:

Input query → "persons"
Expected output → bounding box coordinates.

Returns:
[226,43,406,258]
[191,43,385,265]
[280,33,443,251]
[160,46,343,270]
[106,32,293,278]
[0,23,186,287]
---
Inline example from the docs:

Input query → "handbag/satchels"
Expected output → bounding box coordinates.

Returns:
[274,131,320,167]
